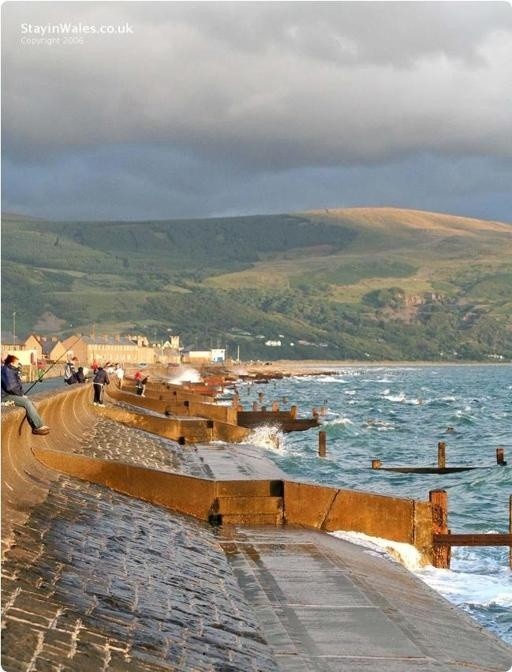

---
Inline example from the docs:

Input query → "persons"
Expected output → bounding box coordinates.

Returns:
[141,375,150,397]
[91,358,99,374]
[92,366,109,407]
[1,354,51,434]
[64,356,88,385]
[135,370,142,388]
[28,352,47,384]
[103,362,124,389]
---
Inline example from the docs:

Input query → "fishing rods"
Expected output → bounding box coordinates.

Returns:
[23,336,83,395]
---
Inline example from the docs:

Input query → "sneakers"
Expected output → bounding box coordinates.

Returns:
[93,402,106,407]
[32,427,52,435]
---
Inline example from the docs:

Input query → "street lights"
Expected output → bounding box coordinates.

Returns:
[160,329,171,357]
[10,311,16,344]
